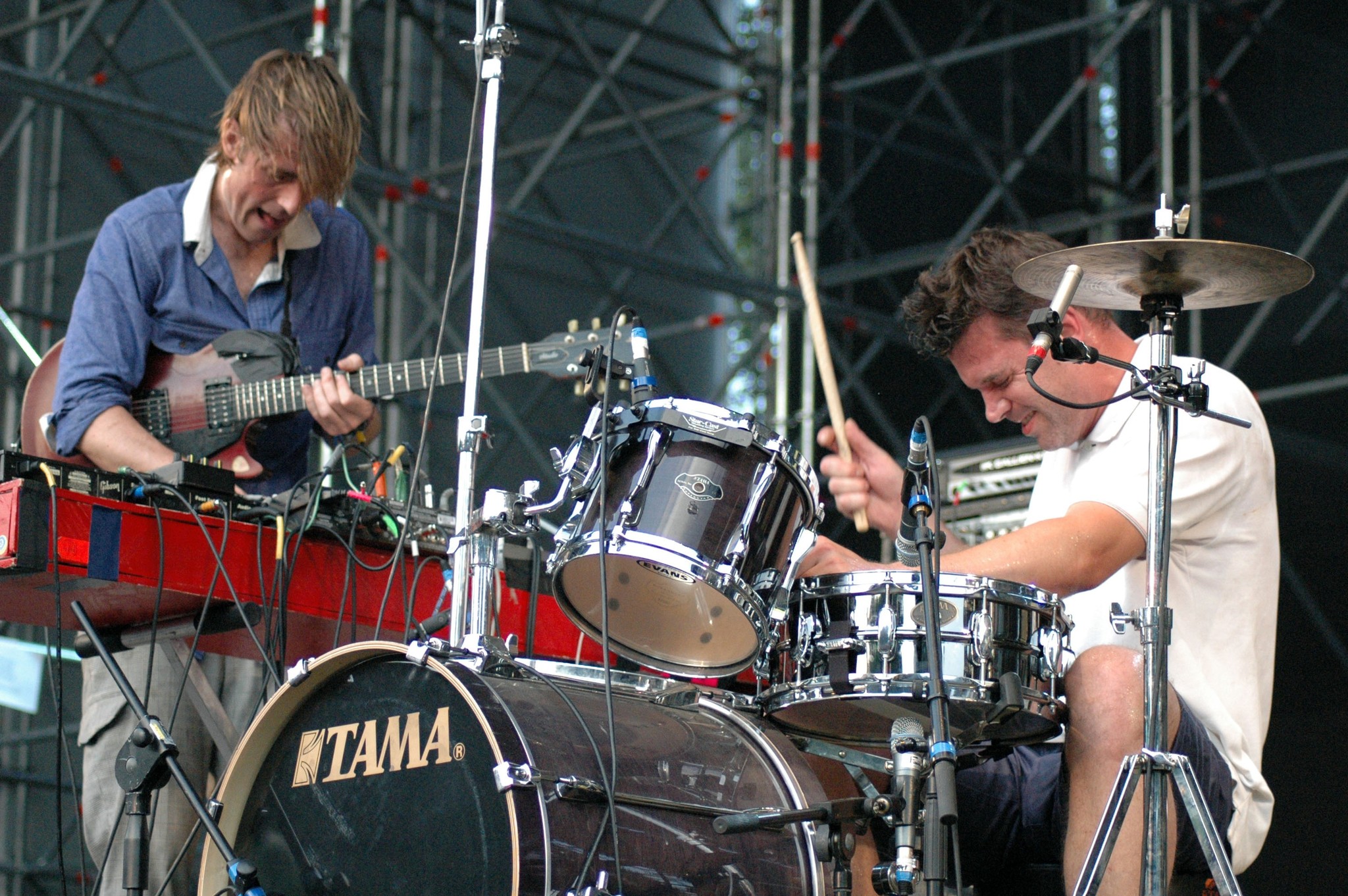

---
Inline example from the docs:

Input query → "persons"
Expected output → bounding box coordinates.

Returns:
[38,46,384,896]
[792,226,1281,896]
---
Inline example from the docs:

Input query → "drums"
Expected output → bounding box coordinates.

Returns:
[553,394,825,682]
[193,636,849,896]
[766,564,1072,748]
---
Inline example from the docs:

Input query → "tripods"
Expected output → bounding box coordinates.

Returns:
[1075,290,1244,896]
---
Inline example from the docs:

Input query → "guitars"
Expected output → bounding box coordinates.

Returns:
[20,308,655,482]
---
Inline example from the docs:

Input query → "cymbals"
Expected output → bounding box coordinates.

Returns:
[1007,235,1319,311]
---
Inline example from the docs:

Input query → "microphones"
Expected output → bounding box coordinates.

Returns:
[628,318,659,405]
[890,716,925,896]
[894,418,929,568]
[1025,264,1084,374]
